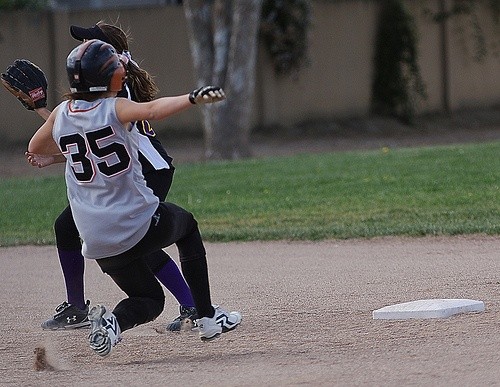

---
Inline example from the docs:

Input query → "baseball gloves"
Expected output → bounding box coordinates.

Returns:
[1,58,49,110]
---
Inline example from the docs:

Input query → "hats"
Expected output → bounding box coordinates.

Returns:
[70,25,117,49]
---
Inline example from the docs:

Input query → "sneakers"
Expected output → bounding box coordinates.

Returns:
[87,305,123,360]
[41,300,91,331]
[166,306,199,333]
[196,305,240,341]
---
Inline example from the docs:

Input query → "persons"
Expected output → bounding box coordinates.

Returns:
[28,39,241,356]
[2,20,198,331]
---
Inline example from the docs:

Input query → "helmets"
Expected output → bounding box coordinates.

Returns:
[66,39,124,91]
